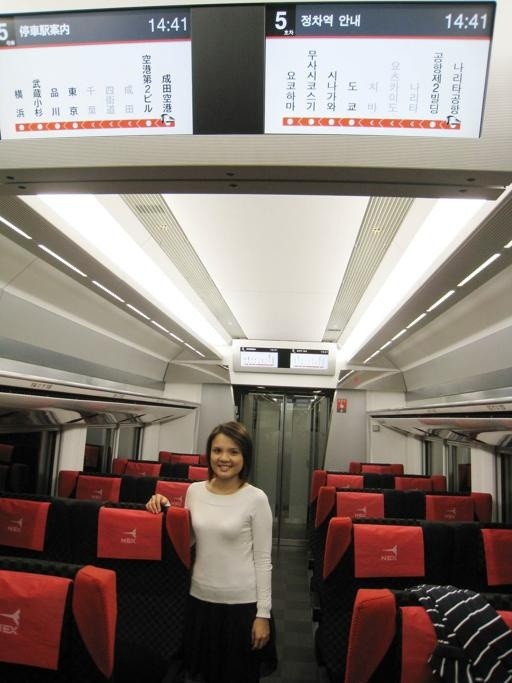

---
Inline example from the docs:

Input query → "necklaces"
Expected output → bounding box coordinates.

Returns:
[204,479,244,495]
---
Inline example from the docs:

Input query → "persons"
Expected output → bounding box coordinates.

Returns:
[143,421,280,683]
